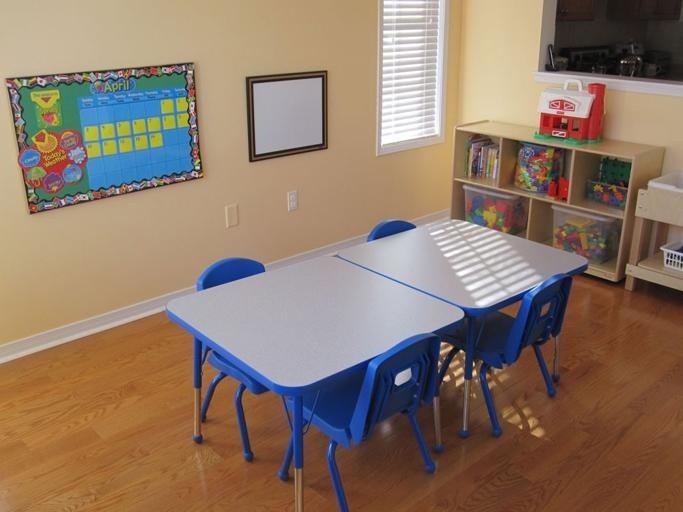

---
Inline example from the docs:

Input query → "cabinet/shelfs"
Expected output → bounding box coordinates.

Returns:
[449,113,664,284]
[623,166,682,296]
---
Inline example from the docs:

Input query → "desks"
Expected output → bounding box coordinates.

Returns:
[164,255,464,511]
[334,217,591,441]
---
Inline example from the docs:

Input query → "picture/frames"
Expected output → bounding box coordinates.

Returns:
[246,69,328,162]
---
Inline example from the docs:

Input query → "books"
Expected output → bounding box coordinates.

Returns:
[464,133,499,183]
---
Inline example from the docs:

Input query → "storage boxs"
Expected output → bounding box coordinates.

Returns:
[460,184,527,237]
[548,203,618,267]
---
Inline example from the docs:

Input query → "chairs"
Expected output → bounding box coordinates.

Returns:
[276,332,437,511]
[193,256,272,464]
[435,274,571,441]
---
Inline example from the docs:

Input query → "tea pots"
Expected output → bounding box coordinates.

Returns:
[644,62,662,75]
